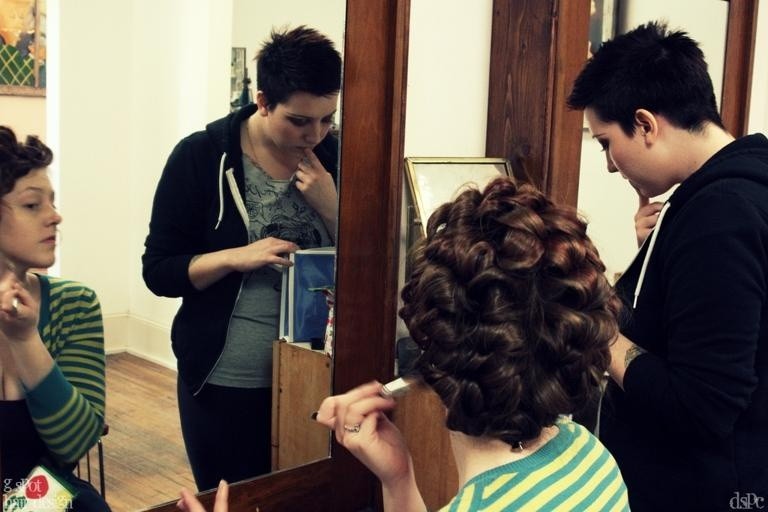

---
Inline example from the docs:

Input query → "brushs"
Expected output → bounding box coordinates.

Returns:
[311,378,425,421]
[5,259,20,315]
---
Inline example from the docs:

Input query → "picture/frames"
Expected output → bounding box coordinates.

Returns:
[405,157,518,239]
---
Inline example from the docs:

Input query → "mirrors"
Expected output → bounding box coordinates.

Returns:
[0,0,409,512]
[546,0,759,289]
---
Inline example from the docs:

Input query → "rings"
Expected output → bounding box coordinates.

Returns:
[344,423,360,432]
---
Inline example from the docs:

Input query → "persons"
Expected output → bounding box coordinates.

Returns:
[564,17,768,512]
[174,176,632,512]
[0,124,111,512]
[141,24,344,491]
